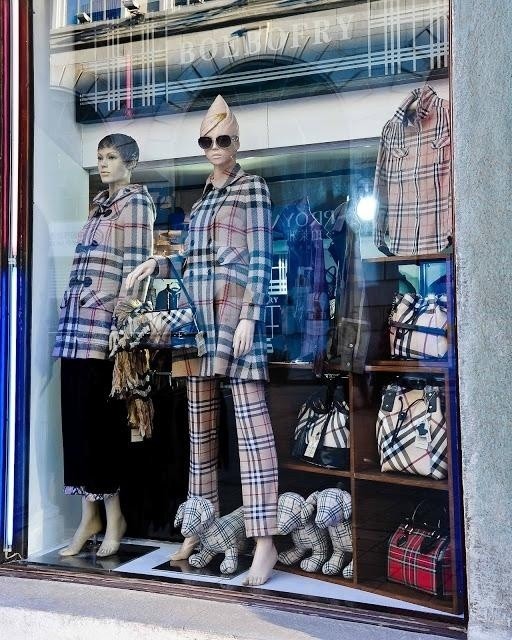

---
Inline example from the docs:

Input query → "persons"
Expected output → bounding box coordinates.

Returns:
[58,132,160,558]
[123,92,281,588]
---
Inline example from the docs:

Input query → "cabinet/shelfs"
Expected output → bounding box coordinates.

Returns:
[254,246,466,617]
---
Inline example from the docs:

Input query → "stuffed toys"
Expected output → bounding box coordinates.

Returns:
[307,487,354,581]
[277,492,332,574]
[174,495,256,576]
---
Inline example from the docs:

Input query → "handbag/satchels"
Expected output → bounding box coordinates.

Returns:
[114,259,207,361]
[386,291,448,360]
[375,376,444,480]
[384,498,452,602]
[289,385,349,469]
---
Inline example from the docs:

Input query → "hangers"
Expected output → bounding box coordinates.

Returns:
[403,75,434,118]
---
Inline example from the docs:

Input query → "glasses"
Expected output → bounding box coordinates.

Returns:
[197,135,233,150]
